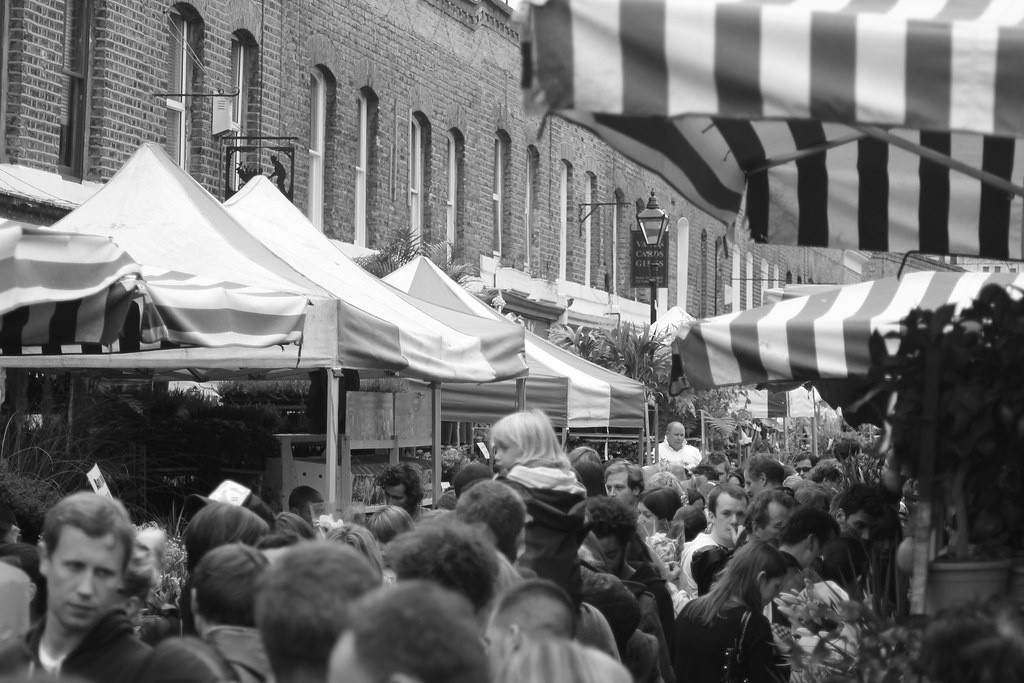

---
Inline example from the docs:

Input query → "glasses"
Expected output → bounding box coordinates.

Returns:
[796,467,811,473]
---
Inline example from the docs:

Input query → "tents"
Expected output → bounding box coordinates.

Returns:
[1,145,649,512]
[513,0,1024,427]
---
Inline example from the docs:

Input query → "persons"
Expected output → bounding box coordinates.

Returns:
[0,410,911,683]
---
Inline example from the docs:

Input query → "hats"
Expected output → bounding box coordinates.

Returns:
[185,480,276,532]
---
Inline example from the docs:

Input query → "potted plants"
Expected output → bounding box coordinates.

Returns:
[849,285,1024,617]
